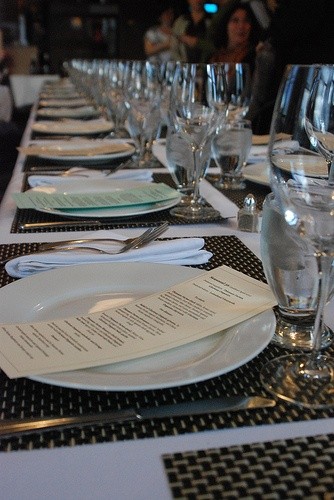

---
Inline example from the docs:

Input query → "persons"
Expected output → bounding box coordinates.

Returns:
[0,0,334,136]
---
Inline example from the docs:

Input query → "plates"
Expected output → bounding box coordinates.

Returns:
[31,181,185,218]
[25,139,135,163]
[0,263,279,392]
[19,77,116,135]
[271,150,333,179]
[241,159,277,187]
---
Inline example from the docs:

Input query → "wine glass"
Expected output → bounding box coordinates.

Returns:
[64,56,333,410]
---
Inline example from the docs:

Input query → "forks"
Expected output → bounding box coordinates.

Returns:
[0,221,170,266]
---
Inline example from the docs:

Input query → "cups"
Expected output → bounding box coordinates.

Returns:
[212,119,254,189]
[256,189,333,350]
[164,113,211,195]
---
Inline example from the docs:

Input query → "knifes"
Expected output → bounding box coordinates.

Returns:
[0,394,275,451]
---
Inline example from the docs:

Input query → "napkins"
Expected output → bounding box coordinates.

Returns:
[28,165,153,188]
[5,237,212,277]
[248,139,299,164]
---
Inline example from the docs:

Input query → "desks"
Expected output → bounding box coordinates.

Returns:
[0,78,334,500]
